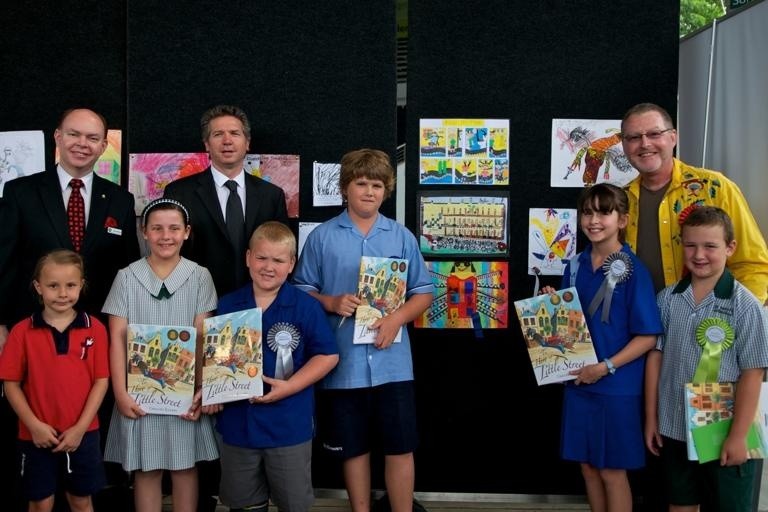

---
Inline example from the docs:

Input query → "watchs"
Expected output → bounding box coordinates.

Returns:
[603,358,616,376]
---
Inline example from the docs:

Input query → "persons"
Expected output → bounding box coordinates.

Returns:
[193,221,339,511]
[0,251,109,512]
[0,109,141,512]
[620,104,768,308]
[646,206,768,512]
[163,108,290,512]
[290,148,432,512]
[536,184,663,512]
[100,201,219,512]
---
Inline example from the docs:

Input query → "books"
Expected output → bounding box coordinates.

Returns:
[126,324,196,416]
[513,289,599,386]
[201,307,264,407]
[353,256,408,345]
[685,384,768,461]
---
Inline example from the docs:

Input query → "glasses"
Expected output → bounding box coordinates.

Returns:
[622,129,673,142]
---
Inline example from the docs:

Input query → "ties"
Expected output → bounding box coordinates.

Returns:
[65,178,86,251]
[224,181,246,247]
